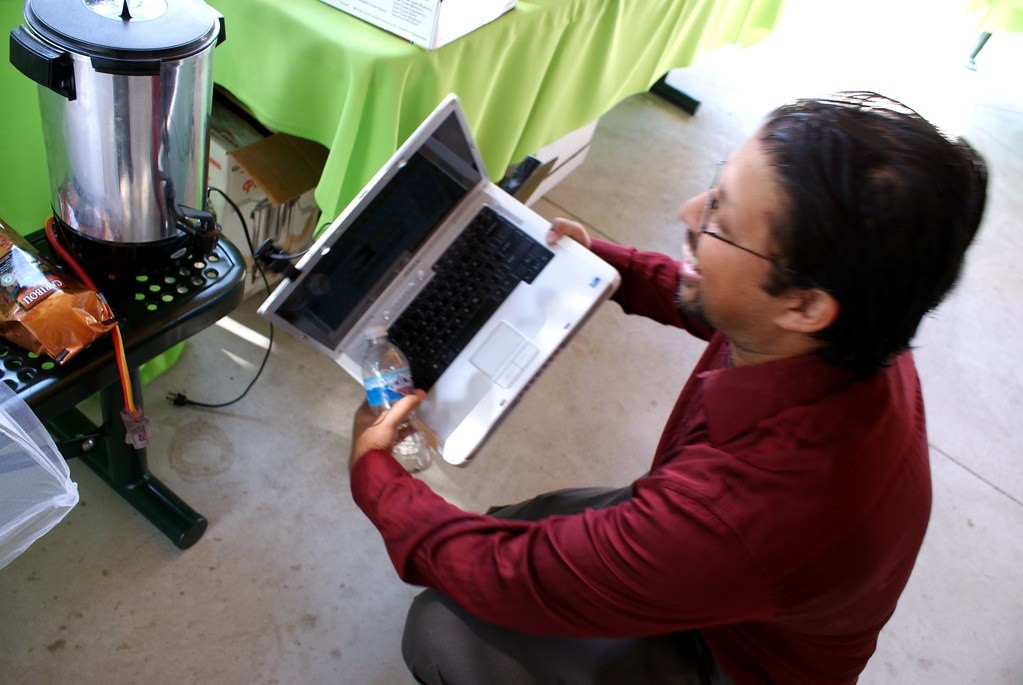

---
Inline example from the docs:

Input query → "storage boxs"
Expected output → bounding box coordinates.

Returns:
[319,0,517,51]
[524,117,598,208]
[203,94,331,302]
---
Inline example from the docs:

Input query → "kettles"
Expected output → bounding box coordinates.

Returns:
[10,0,227,268]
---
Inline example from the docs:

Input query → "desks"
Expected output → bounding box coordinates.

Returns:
[199,0,784,244]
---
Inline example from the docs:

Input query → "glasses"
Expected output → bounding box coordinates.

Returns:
[698,160,792,270]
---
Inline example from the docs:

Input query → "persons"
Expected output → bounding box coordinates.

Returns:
[348,91,991,685]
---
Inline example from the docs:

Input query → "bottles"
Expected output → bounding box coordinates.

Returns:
[363,325,431,476]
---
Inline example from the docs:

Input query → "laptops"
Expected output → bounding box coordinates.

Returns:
[256,93,623,468]
